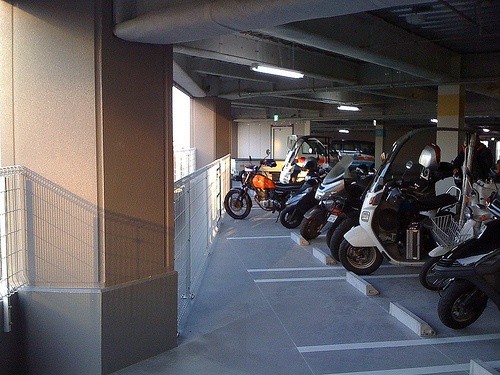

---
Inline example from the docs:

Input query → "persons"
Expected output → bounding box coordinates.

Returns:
[452,142,494,181]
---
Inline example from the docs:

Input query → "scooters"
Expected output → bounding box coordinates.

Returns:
[278,126,500,330]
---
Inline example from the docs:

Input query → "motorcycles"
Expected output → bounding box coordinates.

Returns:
[223,149,309,219]
